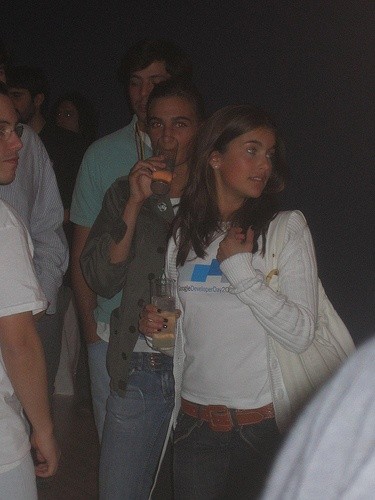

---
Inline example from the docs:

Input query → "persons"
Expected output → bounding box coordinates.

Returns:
[6,67,85,223]
[261,334,375,500]
[78,77,206,500]
[0,81,61,500]
[51,92,97,133]
[67,39,192,444]
[138,105,357,500]
[0,57,68,321]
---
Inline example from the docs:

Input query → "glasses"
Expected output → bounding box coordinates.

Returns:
[0,125,24,141]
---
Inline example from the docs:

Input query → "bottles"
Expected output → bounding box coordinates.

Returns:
[150,136,179,196]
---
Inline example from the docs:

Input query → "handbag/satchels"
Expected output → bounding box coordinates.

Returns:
[268,209,357,432]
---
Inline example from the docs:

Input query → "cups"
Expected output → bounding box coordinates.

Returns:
[150,278,177,351]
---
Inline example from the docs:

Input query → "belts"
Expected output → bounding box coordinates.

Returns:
[131,352,173,370]
[181,396,275,431]
